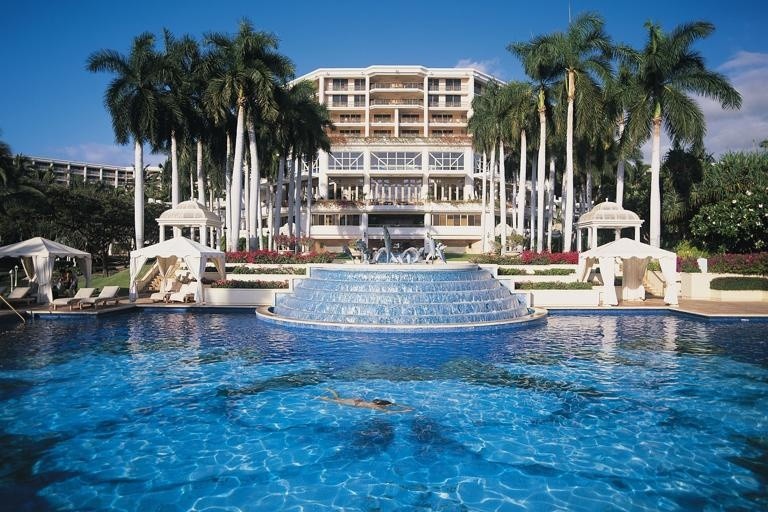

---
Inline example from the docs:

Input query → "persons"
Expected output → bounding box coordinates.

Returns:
[63,270,78,298]
[514,242,524,253]
[303,386,417,412]
[163,274,182,305]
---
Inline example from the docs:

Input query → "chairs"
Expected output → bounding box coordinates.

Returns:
[52,287,96,311]
[78,285,120,310]
[169,281,203,302]
[150,278,179,304]
[0,287,36,308]
